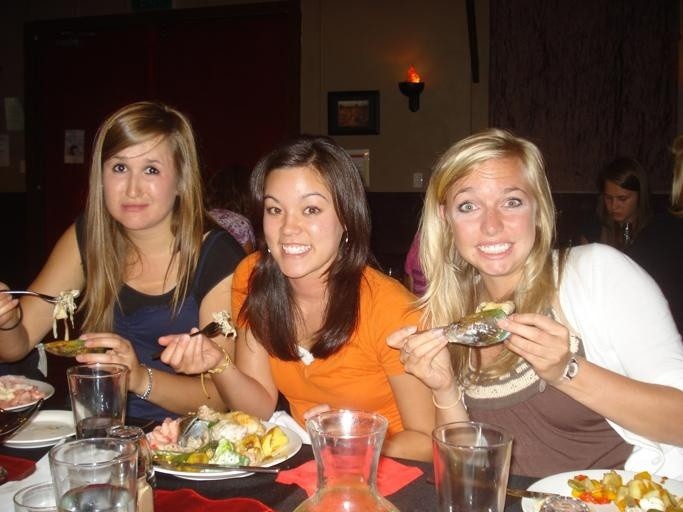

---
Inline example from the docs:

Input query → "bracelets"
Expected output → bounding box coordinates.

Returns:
[432,386,462,409]
[136,363,153,400]
[200,345,236,399]
[1,305,23,331]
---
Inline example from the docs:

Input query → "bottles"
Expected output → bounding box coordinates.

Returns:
[292,408,401,512]
[623,220,633,247]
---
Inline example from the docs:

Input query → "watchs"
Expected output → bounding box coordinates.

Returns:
[553,357,579,388]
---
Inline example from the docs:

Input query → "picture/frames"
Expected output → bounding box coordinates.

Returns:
[326,88,382,137]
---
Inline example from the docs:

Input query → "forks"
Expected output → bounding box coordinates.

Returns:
[152,320,223,361]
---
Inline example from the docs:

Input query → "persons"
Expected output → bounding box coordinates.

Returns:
[0,102,247,423]
[386,129,683,478]
[158,135,435,461]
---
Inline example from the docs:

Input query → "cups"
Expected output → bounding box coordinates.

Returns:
[15,361,155,512]
[431,421,514,512]
[539,494,591,511]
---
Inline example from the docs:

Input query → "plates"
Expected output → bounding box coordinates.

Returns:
[0,376,56,414]
[146,421,304,481]
[522,469,683,512]
[3,409,77,449]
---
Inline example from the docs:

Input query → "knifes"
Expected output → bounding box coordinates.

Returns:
[152,459,283,475]
[427,476,580,501]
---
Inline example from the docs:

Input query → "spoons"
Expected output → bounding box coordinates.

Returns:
[1,289,81,302]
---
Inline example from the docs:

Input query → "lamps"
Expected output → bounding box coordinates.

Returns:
[397,65,426,111]
